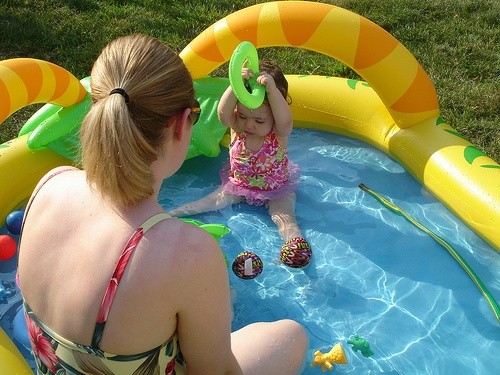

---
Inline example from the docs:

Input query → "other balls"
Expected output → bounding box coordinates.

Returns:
[0,234,17,261]
[6,211,24,235]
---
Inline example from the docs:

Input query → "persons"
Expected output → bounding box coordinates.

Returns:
[16,34,306,375]
[167,57,311,268]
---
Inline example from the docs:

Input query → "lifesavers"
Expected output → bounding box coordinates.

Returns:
[229,41,266,110]
